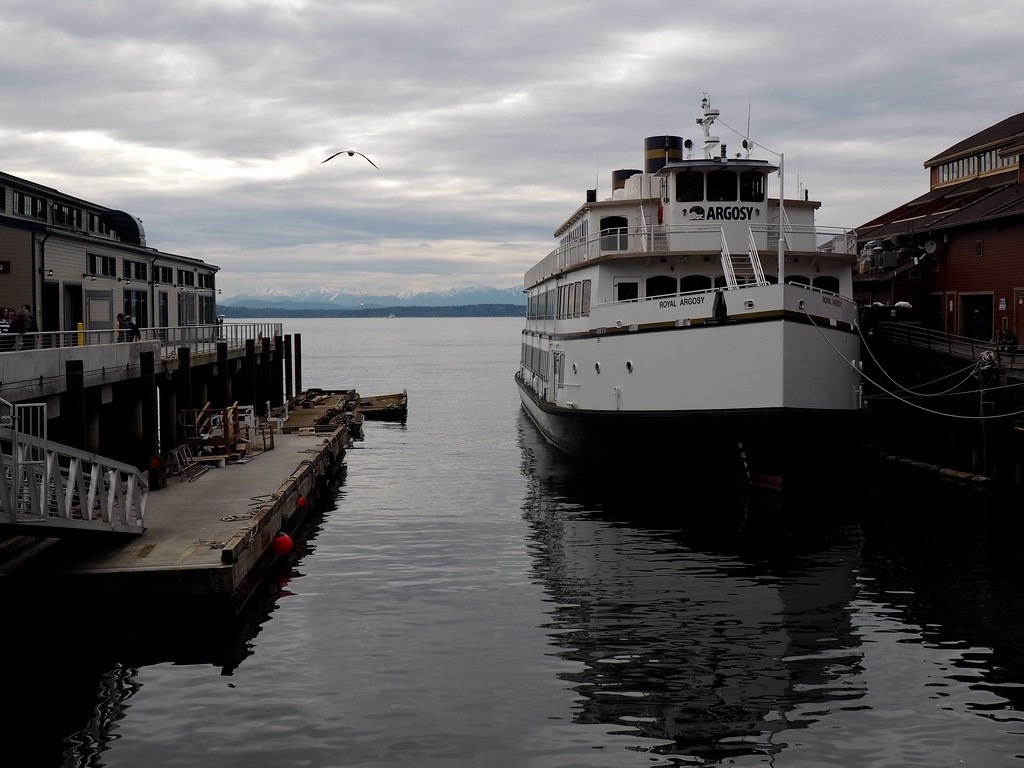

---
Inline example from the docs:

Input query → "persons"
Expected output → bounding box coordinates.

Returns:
[125,315,141,342]
[0,303,40,353]
[116,313,129,343]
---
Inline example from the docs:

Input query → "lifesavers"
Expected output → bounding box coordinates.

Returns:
[657,203,663,224]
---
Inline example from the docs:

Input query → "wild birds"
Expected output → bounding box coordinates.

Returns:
[320,150,380,170]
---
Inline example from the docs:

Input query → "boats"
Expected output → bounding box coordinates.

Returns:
[514,87,863,459]
